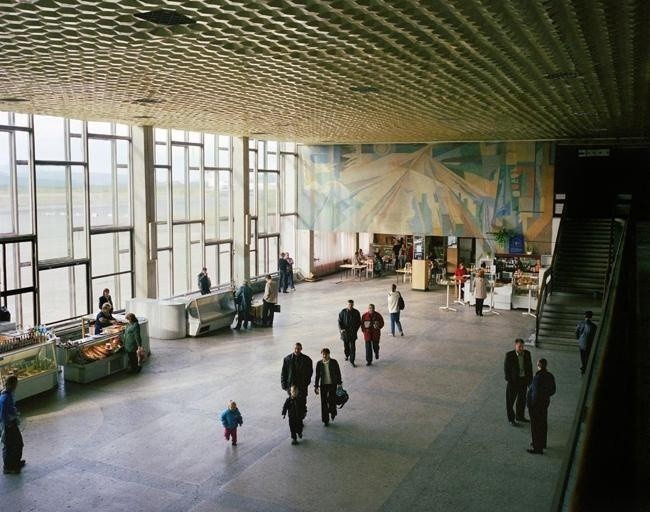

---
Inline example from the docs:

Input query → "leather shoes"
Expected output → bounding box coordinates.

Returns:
[527,442,547,454]
[510,417,530,426]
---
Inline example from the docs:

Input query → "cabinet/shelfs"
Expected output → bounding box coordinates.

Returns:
[496,254,540,285]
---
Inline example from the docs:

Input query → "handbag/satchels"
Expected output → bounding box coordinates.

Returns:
[336,386,348,405]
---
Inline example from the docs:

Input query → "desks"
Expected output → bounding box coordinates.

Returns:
[396,267,412,282]
[338,264,367,281]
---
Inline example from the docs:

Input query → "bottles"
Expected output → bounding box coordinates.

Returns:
[0,322,55,354]
[89,322,95,335]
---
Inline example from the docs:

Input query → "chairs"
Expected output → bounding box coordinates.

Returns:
[403,263,412,282]
[357,255,392,278]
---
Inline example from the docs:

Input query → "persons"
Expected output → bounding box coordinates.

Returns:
[420,248,541,301]
[338,300,361,367]
[197,253,296,330]
[349,235,406,277]
[281,342,313,398]
[526,358,556,454]
[220,399,245,446]
[387,284,404,336]
[0,375,26,475]
[361,303,385,366]
[472,269,490,317]
[281,384,307,445]
[314,347,342,427]
[576,310,596,376]
[94,287,144,374]
[503,337,532,427]
